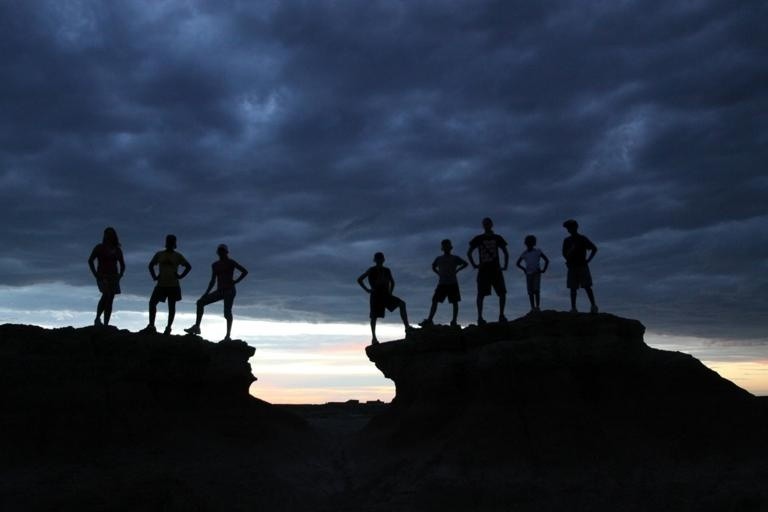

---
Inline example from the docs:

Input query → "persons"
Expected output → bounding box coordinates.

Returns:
[516,235,550,312]
[562,219,599,314]
[137,234,192,334]
[356,251,414,344]
[418,238,468,328]
[184,244,249,344]
[87,226,127,328]
[468,218,509,325]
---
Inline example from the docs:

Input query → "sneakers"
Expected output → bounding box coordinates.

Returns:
[184,325,201,334]
[95,318,103,325]
[219,336,233,345]
[450,320,457,326]
[405,325,420,332]
[372,338,379,345]
[140,324,156,333]
[590,304,598,312]
[418,319,433,327]
[499,314,507,322]
[478,319,486,326]
[164,327,171,335]
[527,306,540,315]
